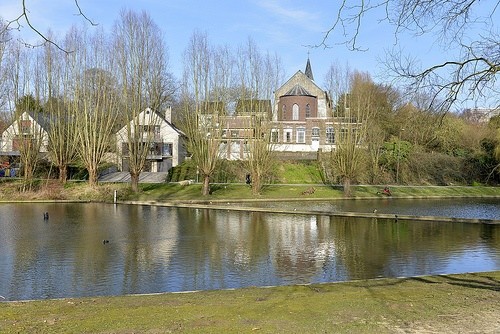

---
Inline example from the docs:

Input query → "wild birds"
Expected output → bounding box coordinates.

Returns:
[103,240,109,245]
[373,208,377,213]
[394,215,399,218]
[43,211,50,221]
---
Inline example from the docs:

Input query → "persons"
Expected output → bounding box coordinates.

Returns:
[246,172,251,186]
[384,186,392,196]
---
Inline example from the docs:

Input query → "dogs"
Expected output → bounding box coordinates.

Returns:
[299,186,318,196]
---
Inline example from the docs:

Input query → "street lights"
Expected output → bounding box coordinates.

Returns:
[395,128,404,186]
